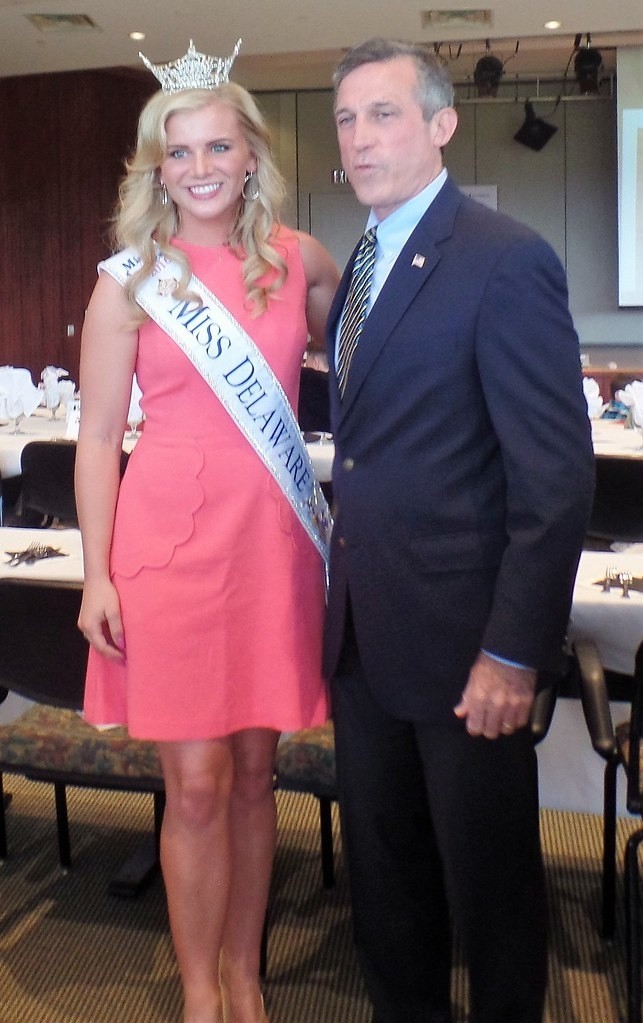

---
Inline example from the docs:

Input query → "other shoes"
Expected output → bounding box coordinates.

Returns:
[217,947,268,1023]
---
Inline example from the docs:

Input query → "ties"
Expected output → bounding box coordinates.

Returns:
[337,224,378,401]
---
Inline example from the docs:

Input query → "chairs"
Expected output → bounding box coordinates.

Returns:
[574,638,632,949]
[19,440,128,530]
[260,721,339,978]
[0,702,167,898]
[624,640,643,1023]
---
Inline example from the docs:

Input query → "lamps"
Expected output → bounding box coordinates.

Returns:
[573,47,603,91]
[472,57,505,98]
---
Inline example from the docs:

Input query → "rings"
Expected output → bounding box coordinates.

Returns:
[503,722,515,731]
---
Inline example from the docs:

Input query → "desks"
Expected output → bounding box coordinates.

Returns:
[0,408,643,551]
[0,525,643,854]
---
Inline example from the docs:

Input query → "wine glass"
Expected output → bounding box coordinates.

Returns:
[46,396,61,420]
[7,401,27,435]
[38,382,47,406]
[127,406,142,439]
[631,409,643,450]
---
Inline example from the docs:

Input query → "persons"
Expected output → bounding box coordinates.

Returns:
[73,36,341,1023]
[321,37,598,1023]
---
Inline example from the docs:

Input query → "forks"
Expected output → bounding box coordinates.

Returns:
[602,565,632,598]
[4,542,44,567]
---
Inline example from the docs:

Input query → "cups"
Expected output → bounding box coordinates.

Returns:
[580,354,589,371]
